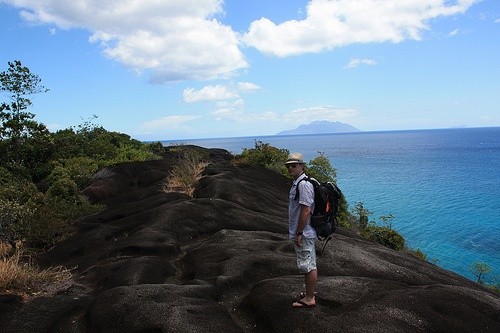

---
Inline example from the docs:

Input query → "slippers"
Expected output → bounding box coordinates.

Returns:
[300,291,317,302]
[291,300,317,308]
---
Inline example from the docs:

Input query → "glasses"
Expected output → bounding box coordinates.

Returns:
[285,163,297,169]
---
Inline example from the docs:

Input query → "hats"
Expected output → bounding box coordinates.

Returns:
[284,152,307,163]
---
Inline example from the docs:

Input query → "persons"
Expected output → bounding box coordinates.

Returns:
[284,153,318,307]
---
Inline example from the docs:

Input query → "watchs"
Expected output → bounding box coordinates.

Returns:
[296,232,303,235]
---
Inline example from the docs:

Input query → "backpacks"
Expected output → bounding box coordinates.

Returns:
[293,173,341,241]
